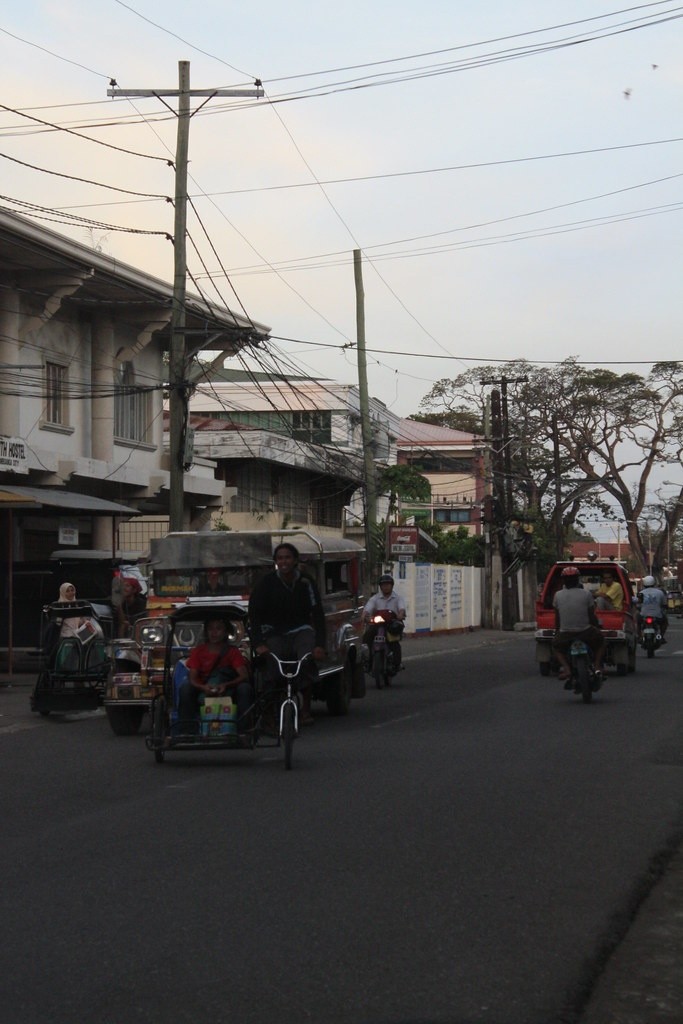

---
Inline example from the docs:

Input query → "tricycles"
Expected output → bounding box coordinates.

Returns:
[143,595,317,770]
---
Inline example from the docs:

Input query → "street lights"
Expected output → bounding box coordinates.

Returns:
[625,520,652,576]
[641,510,672,588]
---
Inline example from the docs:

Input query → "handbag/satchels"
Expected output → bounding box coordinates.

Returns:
[198,669,237,705]
[200,696,238,736]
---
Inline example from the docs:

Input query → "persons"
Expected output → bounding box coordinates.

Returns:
[360,575,406,673]
[115,577,148,639]
[549,566,668,680]
[54,582,88,674]
[247,543,327,734]
[178,618,255,741]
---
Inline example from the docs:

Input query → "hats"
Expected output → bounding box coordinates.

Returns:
[125,577,142,593]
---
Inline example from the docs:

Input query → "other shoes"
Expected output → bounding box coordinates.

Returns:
[638,637,643,644]
[360,654,369,665]
[558,671,571,680]
[660,638,667,644]
[238,716,253,731]
[392,664,401,672]
[254,692,267,707]
[300,711,314,727]
[184,721,198,735]
[592,667,609,676]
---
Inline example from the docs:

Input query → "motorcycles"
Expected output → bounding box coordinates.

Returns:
[365,610,405,690]
[562,627,603,705]
[640,615,663,660]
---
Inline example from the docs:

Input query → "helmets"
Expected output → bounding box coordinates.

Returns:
[643,576,655,586]
[390,620,404,635]
[378,575,394,586]
[560,566,580,577]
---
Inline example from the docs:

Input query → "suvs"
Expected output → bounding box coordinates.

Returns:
[99,528,366,739]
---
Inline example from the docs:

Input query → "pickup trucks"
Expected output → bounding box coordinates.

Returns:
[534,550,644,679]
